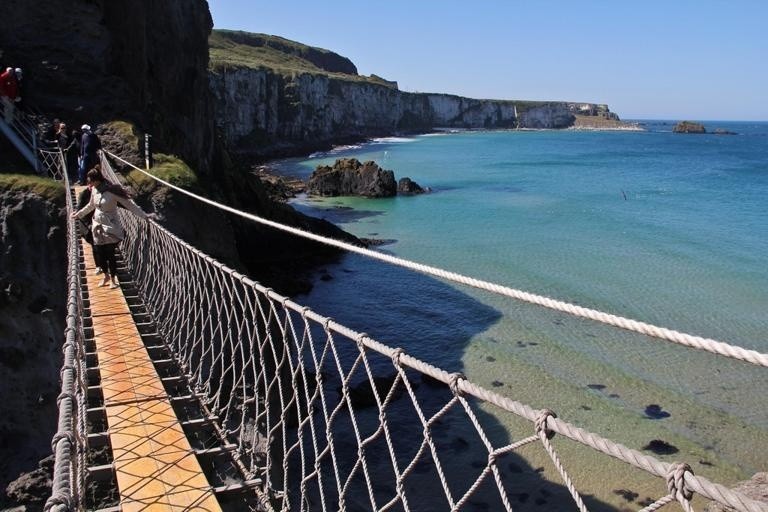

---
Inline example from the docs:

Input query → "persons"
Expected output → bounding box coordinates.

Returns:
[70,163,157,291]
[0,65,19,129]
[12,67,27,122]
[74,178,102,276]
[36,116,102,186]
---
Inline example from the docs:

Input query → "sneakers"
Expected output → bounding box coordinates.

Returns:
[94,268,119,289]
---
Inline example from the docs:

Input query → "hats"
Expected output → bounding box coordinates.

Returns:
[16,68,21,72]
[7,67,13,72]
[81,123,90,130]
